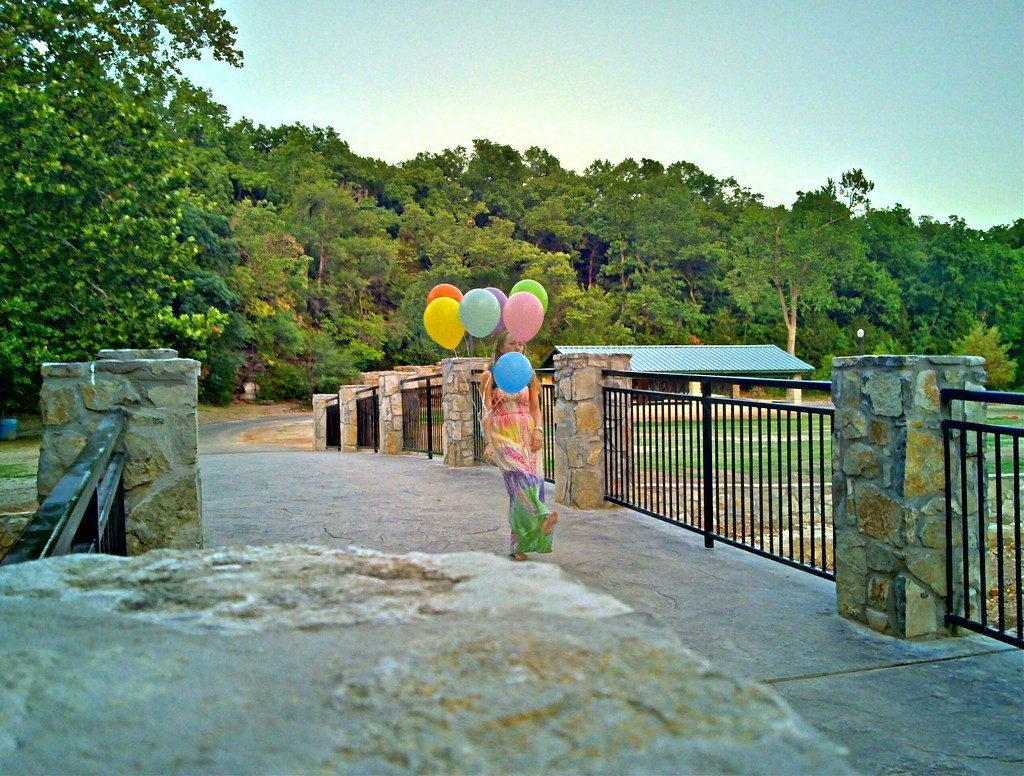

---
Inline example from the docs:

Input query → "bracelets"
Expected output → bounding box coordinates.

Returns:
[534,427,543,433]
[485,442,492,446]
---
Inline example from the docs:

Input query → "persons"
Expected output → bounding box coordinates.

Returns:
[483,331,558,560]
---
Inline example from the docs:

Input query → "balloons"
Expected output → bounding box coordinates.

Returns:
[484,287,508,336]
[459,289,500,338]
[509,279,548,314]
[493,352,532,394]
[503,292,545,344]
[426,284,464,305]
[423,297,464,349]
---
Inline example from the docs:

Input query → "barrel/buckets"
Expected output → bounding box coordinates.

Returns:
[0,418,19,441]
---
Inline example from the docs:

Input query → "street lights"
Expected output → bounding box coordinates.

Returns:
[856,328,865,356]
